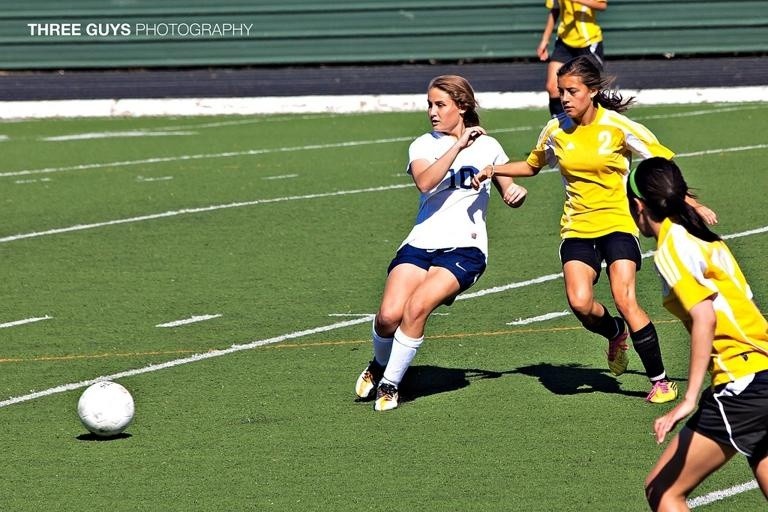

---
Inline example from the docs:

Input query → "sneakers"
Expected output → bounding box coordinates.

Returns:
[607,315,629,376]
[647,378,678,404]
[374,378,399,412]
[355,355,383,399]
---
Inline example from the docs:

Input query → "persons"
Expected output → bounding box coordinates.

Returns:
[536,0,609,116]
[357,54,718,412]
[626,153,768,512]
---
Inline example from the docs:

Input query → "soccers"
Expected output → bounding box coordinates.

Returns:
[77,380,135,436]
